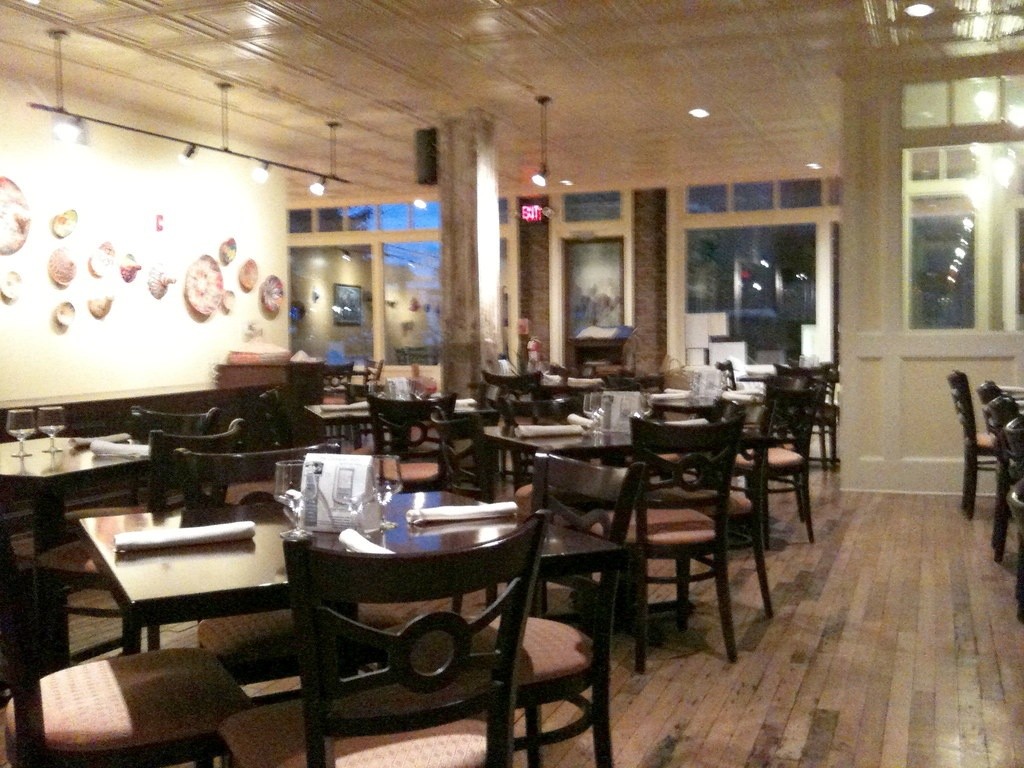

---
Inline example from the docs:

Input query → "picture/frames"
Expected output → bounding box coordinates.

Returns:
[334,283,362,325]
[558,233,627,338]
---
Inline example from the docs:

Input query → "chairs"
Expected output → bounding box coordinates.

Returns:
[947,369,1024,626]
[0,358,843,768]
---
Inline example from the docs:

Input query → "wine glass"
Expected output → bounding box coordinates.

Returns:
[368,378,396,400]
[583,392,609,435]
[6,408,36,457]
[408,377,436,399]
[273,459,320,541]
[369,453,402,529]
[621,391,653,418]
[36,407,65,452]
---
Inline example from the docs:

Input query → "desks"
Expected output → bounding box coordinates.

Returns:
[0,436,152,662]
[76,489,628,655]
[650,396,719,414]
[483,426,639,461]
[307,398,494,449]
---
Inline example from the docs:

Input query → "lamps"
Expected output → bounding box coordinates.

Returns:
[57,112,84,142]
[311,175,329,198]
[335,247,352,262]
[175,145,198,165]
[529,95,552,187]
[247,161,272,185]
